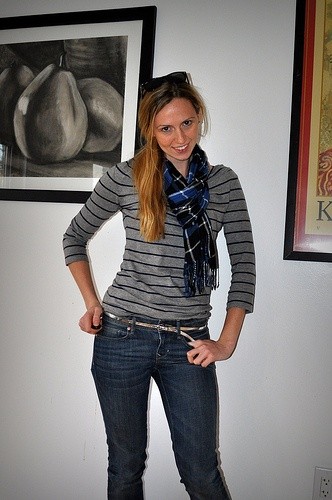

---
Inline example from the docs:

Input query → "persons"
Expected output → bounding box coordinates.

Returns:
[61,70,257,500]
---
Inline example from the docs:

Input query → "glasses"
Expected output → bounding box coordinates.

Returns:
[140,70,190,99]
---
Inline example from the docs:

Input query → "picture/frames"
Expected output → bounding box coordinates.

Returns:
[0,6,157,204]
[283,0,332,262]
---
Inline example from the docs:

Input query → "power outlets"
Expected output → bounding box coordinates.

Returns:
[312,467,332,500]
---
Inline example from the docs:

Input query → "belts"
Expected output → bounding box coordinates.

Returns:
[104,311,208,342]
